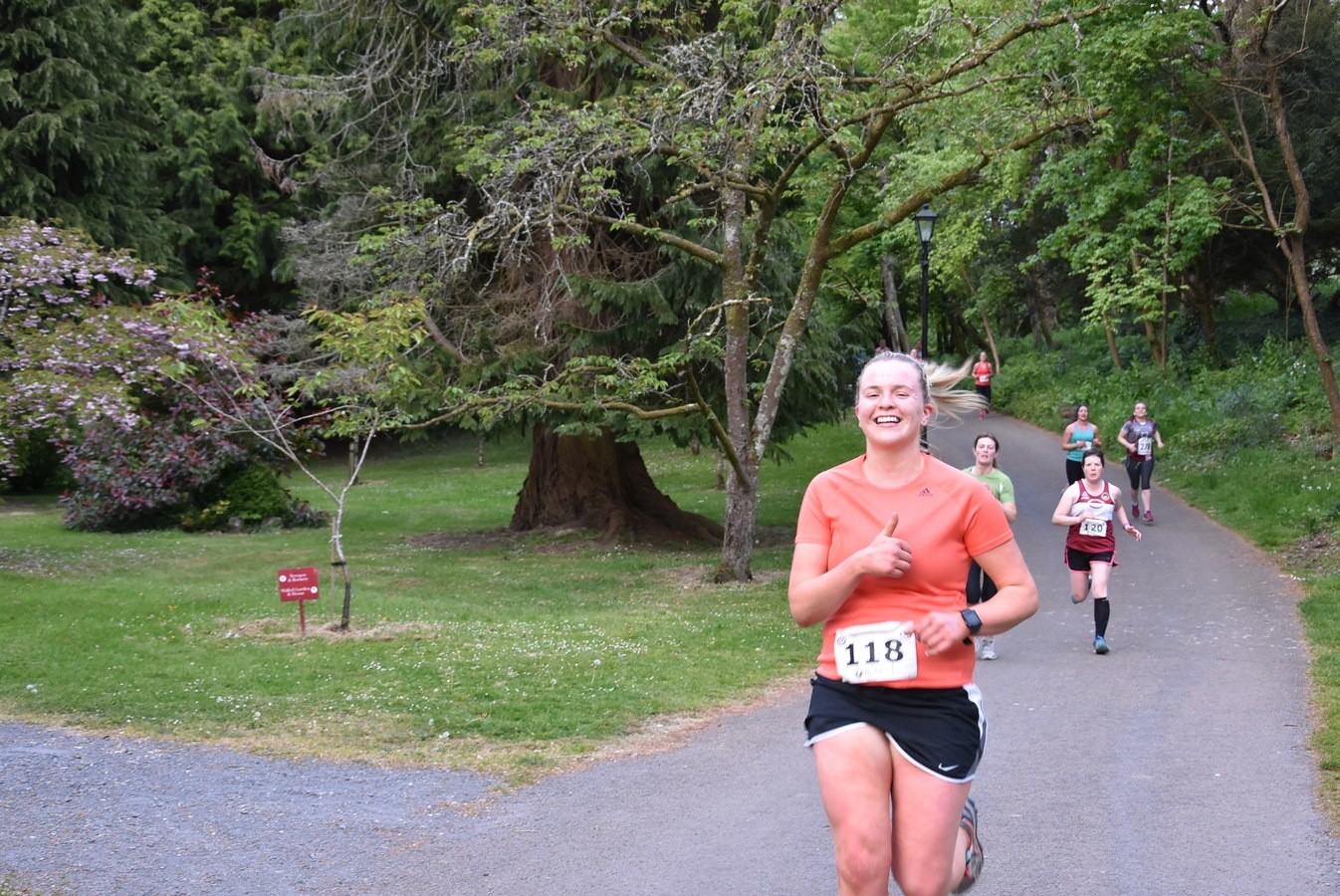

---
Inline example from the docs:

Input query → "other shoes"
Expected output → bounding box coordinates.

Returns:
[983,409,988,414]
[974,638,980,653]
[979,414,984,419]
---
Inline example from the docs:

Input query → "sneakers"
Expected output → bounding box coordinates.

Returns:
[981,637,997,660]
[951,795,983,894]
[1143,511,1153,522]
[1071,575,1093,605]
[1131,504,1140,518]
[1093,636,1110,655]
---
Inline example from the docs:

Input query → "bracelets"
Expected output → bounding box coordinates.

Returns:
[1122,523,1133,530]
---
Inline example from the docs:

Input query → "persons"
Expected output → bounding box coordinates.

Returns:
[1116,402,1165,522]
[1061,405,1102,497]
[959,434,1018,659]
[1050,449,1143,656]
[874,340,926,364]
[972,351,995,419]
[787,351,1039,896]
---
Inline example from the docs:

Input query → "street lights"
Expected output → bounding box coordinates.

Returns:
[911,203,940,456]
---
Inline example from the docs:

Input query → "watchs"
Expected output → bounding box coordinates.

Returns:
[960,610,982,636]
